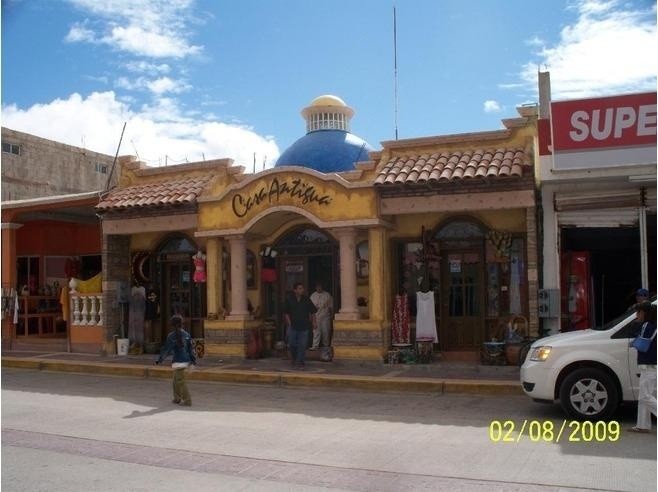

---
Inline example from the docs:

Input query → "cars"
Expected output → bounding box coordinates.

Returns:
[516,297,656,424]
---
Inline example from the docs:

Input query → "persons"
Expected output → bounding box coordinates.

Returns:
[628,301,657,433]
[309,284,334,351]
[635,288,648,302]
[192,250,207,284]
[152,314,197,407]
[282,282,318,368]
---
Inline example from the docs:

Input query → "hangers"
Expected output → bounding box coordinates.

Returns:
[416,283,434,294]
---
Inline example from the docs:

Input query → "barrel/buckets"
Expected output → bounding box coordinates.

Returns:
[263,327,277,353]
[116,338,130,355]
[482,342,506,365]
[503,341,523,363]
